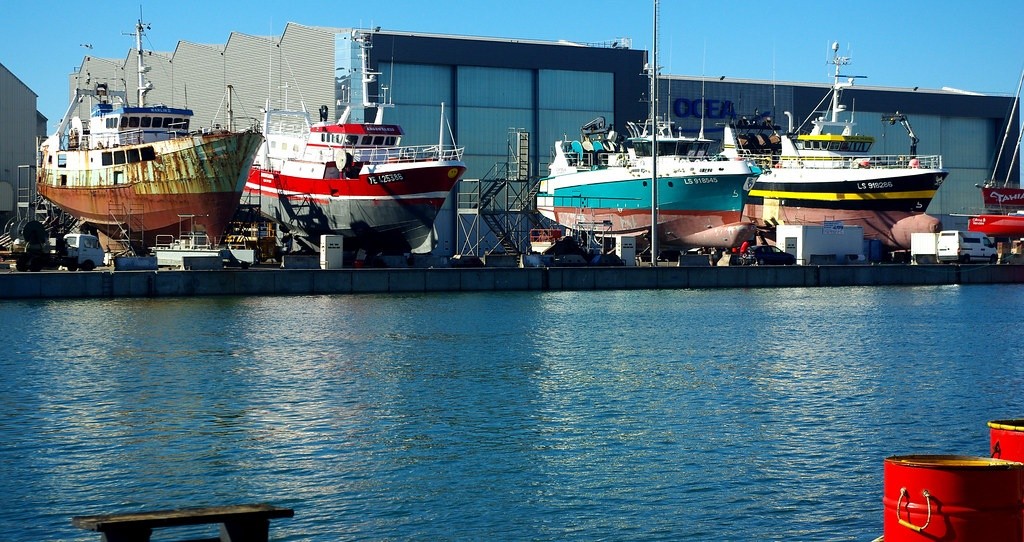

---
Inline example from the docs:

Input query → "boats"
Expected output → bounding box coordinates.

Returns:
[234,18,466,255]
[734,39,951,253]
[533,115,760,252]
[32,2,266,248]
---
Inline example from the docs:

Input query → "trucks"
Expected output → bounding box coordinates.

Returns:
[940,230,999,262]
[0,233,105,269]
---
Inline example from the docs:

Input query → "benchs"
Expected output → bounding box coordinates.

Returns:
[71,504,295,542]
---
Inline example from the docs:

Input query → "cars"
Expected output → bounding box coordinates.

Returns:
[742,244,796,265]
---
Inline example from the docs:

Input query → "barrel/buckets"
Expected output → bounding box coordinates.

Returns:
[986,420,1024,464]
[883,454,1024,542]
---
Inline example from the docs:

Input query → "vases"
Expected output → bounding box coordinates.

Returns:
[354,259,364,268]
